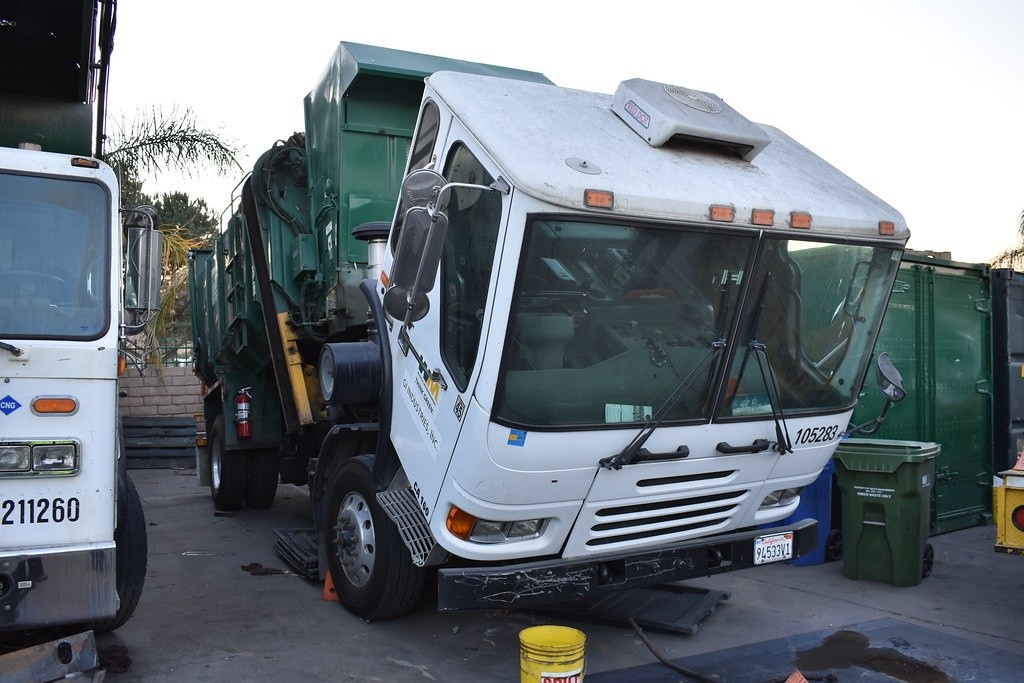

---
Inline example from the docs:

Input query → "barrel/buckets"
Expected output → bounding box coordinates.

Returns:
[519,625,587,683]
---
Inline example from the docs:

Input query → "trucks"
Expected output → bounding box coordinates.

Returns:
[0,3,164,681]
[181,42,912,626]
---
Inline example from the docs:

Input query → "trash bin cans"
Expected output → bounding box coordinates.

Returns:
[756,446,835,567]
[834,438,940,587]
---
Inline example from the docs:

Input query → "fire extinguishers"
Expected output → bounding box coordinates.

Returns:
[235,387,252,438]
